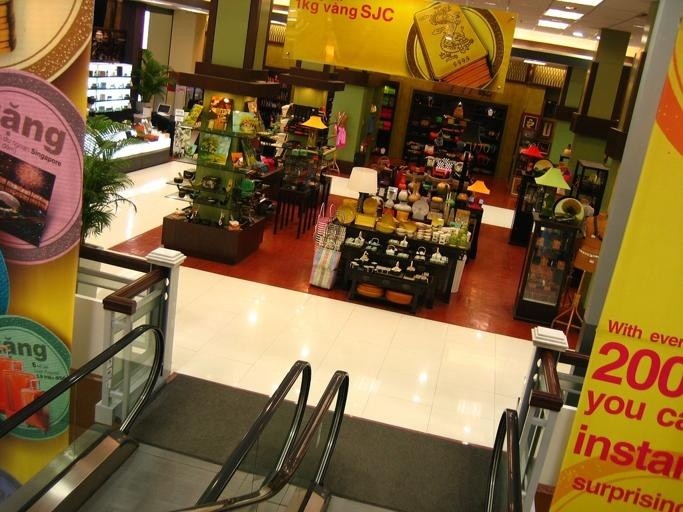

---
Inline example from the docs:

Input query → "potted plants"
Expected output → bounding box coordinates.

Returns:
[134,50,176,119]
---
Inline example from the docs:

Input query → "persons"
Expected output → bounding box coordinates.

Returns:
[91,26,119,62]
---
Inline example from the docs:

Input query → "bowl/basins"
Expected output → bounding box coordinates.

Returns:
[174,177,183,183]
[440,227,471,242]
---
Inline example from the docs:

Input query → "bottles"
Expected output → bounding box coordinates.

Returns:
[0,355,50,430]
[354,231,364,247]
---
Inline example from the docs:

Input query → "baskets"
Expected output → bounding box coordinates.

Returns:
[313,202,336,258]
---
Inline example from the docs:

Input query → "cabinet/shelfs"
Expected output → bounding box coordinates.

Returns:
[335,150,465,314]
[570,160,608,216]
[405,89,510,181]
[87,58,138,123]
[158,101,265,267]
[513,217,577,326]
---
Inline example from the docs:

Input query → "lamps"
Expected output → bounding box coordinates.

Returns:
[466,177,490,210]
[520,144,542,174]
[299,116,326,149]
[533,168,571,219]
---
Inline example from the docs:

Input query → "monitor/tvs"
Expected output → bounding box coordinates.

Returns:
[157,103,171,113]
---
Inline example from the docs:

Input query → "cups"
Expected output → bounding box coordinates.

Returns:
[178,190,184,198]
[447,223,470,246]
[393,229,450,245]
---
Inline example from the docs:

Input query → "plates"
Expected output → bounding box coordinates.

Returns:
[335,205,355,225]
[356,282,412,305]
[405,4,503,90]
